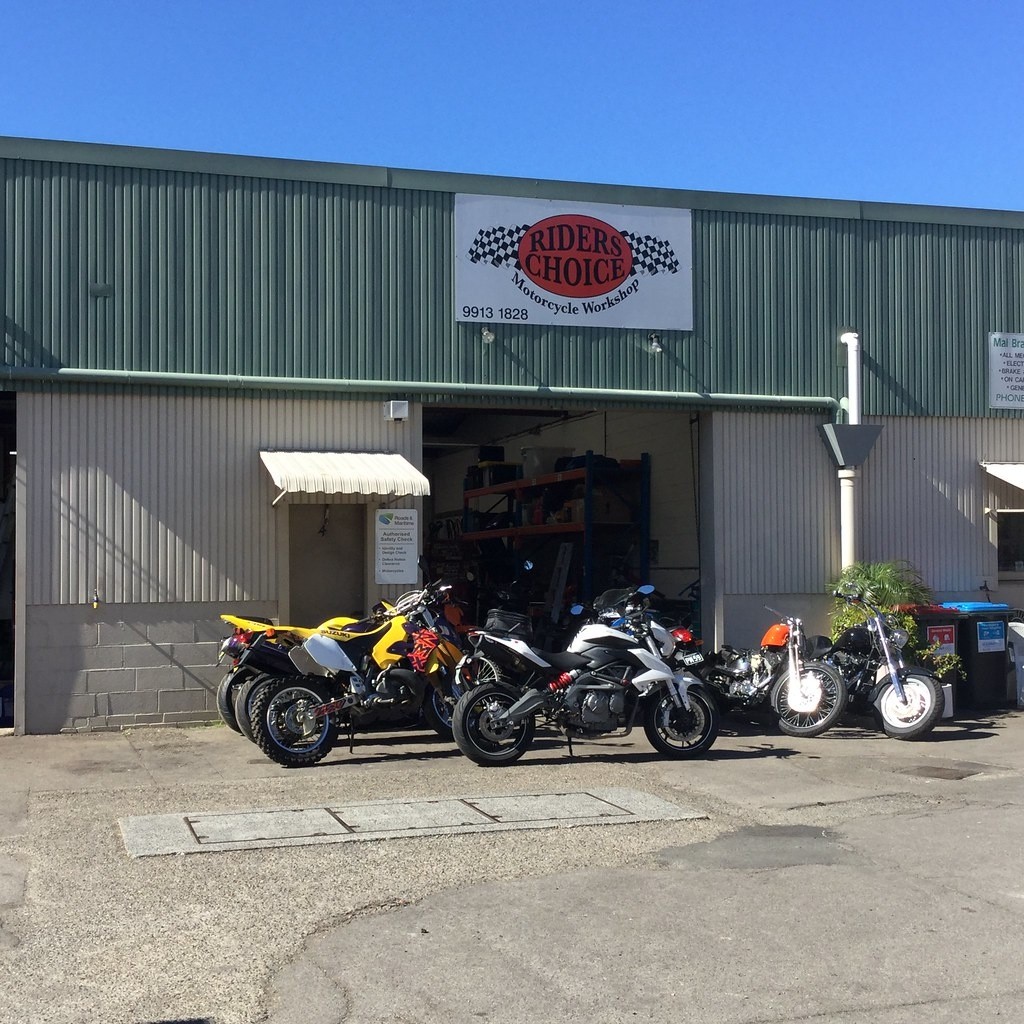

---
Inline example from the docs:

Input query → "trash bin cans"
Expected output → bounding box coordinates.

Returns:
[890,603,969,726]
[939,601,1010,712]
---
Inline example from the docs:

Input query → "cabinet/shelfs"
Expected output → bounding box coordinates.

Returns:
[461,450,651,625]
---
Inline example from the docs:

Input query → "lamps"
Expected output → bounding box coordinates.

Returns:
[649,333,662,352]
[481,328,495,343]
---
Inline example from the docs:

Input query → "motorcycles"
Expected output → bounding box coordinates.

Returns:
[214,553,945,768]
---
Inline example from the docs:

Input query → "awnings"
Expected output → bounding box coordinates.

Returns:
[260,450,431,496]
[980,461,1024,490]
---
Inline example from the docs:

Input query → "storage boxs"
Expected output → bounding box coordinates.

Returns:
[463,445,523,491]
[572,494,608,522]
